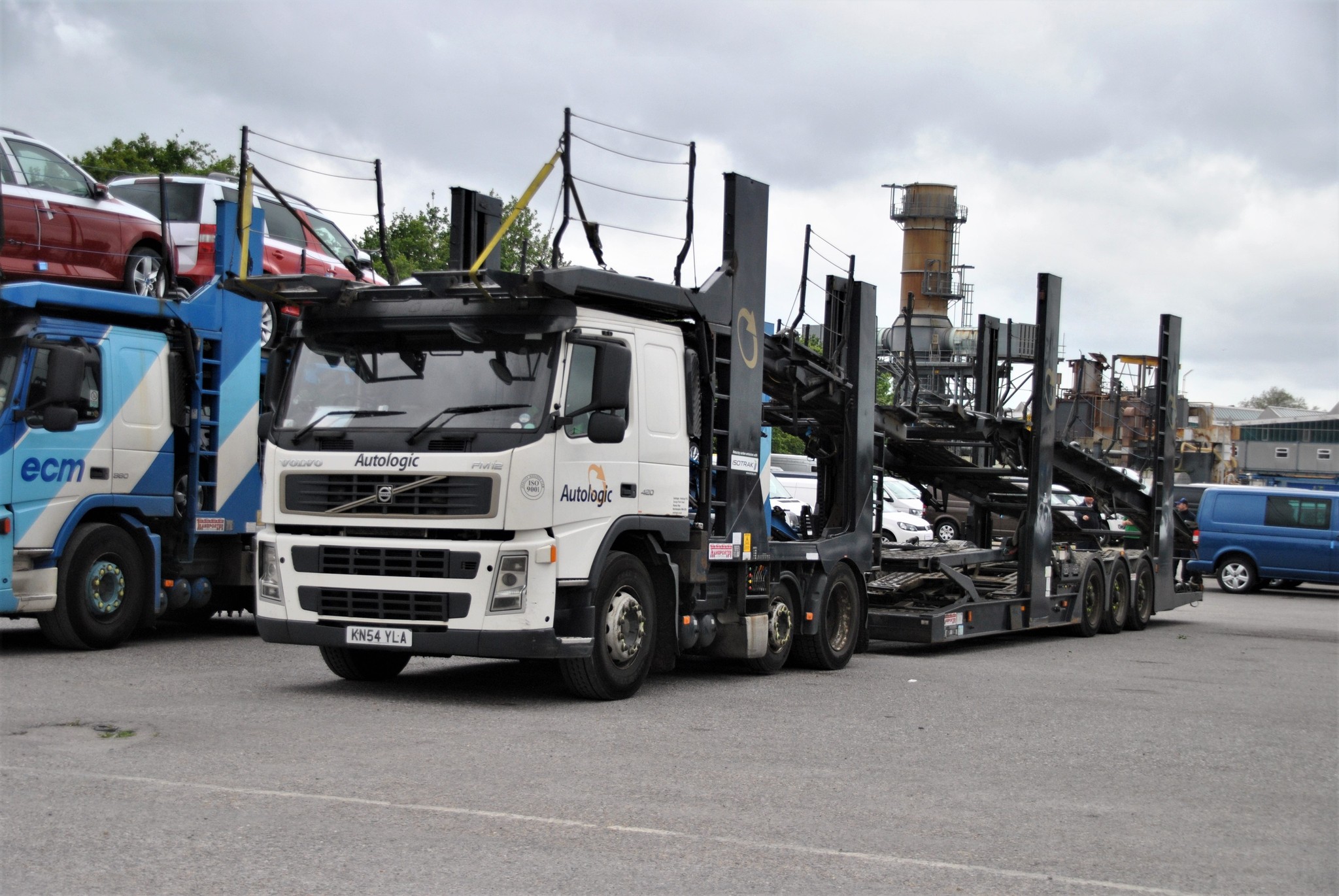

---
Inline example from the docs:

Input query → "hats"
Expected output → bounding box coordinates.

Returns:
[1174,498,1188,504]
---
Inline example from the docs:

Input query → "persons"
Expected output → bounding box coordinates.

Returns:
[1172,498,1196,583]
[1223,470,1237,485]
[1122,516,1144,550]
[1074,496,1102,552]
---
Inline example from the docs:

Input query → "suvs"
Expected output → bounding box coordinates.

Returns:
[0,125,181,302]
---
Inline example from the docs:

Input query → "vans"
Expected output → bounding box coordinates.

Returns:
[773,475,935,545]
[106,172,393,350]
[920,483,1023,542]
[1185,484,1339,594]
[874,476,926,519]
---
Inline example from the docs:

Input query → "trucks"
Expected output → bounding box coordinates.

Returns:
[221,104,1205,702]
[1,198,797,653]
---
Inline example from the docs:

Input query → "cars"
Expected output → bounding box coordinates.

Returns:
[709,453,813,515]
[1050,466,1149,533]
